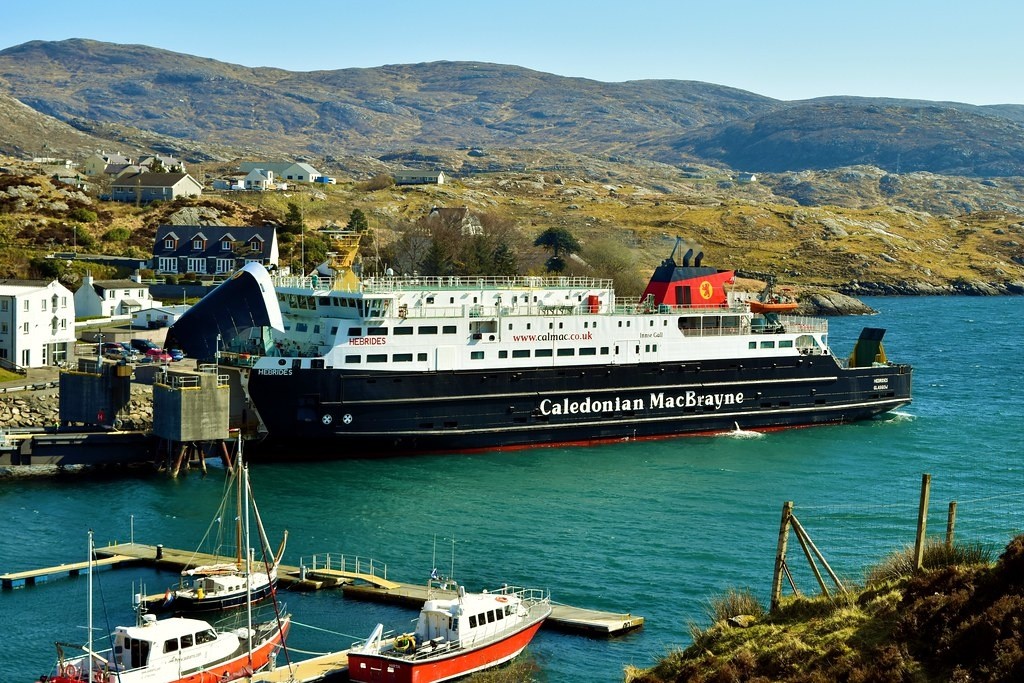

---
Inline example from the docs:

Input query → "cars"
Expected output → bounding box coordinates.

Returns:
[97,342,140,363]
[137,347,184,366]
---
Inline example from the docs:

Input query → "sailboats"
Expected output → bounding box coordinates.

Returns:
[136,430,289,621]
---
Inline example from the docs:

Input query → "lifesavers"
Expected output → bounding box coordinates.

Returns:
[66,663,76,680]
[496,593,508,604]
[96,670,106,682]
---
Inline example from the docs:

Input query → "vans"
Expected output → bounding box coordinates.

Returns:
[129,338,159,354]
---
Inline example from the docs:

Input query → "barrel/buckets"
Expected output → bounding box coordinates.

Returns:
[752,319,765,332]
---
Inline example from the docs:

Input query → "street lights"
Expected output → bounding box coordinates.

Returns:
[129,319,134,338]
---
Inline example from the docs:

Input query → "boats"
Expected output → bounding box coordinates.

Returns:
[37,463,292,683]
[248,186,913,459]
[346,533,552,683]
[745,296,800,314]
